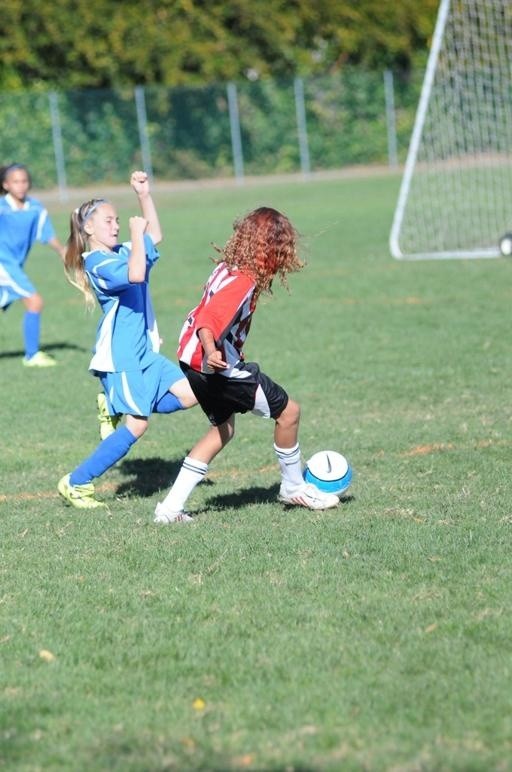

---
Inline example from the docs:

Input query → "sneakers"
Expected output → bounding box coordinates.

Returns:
[21,350,61,367]
[96,391,119,441]
[279,481,339,509]
[153,501,195,526]
[57,474,107,510]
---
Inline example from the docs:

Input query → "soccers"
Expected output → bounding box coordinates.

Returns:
[304,451,352,496]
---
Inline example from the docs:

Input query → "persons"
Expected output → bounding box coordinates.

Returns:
[57,171,201,511]
[151,206,339,528]
[0,161,69,367]
